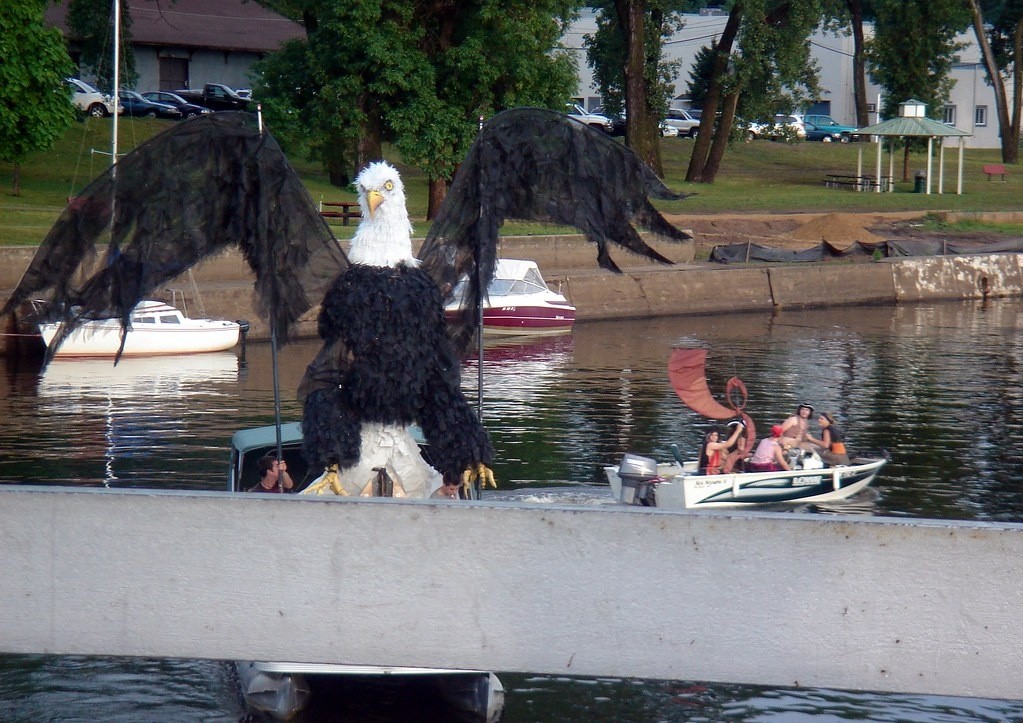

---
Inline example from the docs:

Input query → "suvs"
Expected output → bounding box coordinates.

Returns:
[554,103,615,140]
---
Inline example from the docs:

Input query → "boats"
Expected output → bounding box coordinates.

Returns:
[603,441,888,510]
[432,257,579,340]
[599,453,895,514]
[226,419,514,723]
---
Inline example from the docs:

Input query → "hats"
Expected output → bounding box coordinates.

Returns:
[798,400,815,411]
[771,424,783,437]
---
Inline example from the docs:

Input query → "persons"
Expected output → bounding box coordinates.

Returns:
[248,456,293,493]
[780,402,814,451]
[749,424,791,471]
[429,469,461,500]
[698,423,747,475]
[806,411,841,468]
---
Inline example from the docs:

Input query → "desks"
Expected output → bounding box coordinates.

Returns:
[826,174,888,192]
[323,201,360,225]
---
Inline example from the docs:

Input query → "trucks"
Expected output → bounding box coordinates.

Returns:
[161,83,244,111]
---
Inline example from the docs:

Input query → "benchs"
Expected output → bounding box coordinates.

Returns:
[320,210,362,217]
[984,164,1006,181]
[822,179,891,192]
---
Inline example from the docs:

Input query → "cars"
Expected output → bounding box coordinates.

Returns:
[589,104,864,144]
[102,89,183,120]
[140,90,215,119]
[235,88,255,101]
[52,77,126,118]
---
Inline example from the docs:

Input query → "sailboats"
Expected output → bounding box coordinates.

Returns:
[36,0,242,359]
